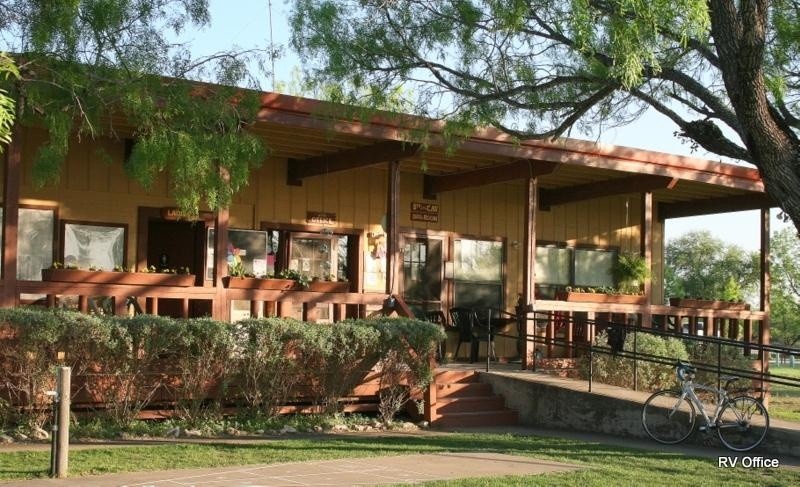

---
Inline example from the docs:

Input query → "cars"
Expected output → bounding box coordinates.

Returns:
[682,321,720,339]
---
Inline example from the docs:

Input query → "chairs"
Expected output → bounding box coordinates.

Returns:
[450,308,498,363]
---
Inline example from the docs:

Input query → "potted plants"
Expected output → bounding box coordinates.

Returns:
[670,295,746,310]
[558,285,648,305]
[41,261,197,287]
[222,262,351,292]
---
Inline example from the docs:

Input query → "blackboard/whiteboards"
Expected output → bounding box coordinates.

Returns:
[205,227,268,281]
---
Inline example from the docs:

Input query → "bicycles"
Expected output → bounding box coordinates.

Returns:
[641,359,769,453]
